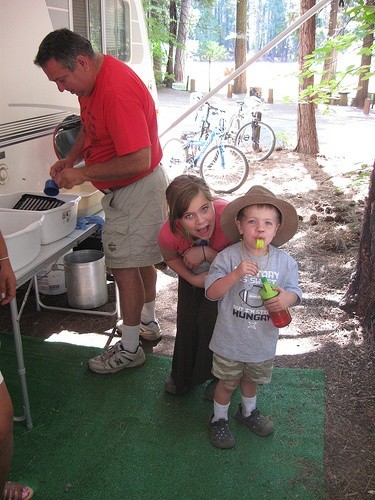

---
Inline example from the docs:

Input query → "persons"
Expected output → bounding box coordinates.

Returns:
[204,184,303,450]
[0,232,34,500]
[155,174,230,398]
[33,27,169,375]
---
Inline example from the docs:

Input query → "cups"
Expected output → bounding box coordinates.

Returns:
[44,179,59,196]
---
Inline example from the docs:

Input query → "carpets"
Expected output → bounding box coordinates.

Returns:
[0,329,325,500]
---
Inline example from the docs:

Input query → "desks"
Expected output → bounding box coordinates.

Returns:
[0,209,121,430]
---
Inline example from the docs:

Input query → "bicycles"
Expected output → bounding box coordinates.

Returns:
[161,96,276,194]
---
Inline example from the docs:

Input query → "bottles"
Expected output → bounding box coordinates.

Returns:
[259,277,292,327]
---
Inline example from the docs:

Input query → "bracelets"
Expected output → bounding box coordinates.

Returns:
[0,256,9,261]
[202,246,206,262]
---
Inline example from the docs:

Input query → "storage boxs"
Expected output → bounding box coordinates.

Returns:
[0,181,106,273]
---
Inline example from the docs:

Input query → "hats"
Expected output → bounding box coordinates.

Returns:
[220,185,298,248]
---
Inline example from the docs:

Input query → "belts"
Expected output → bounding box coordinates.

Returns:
[101,185,123,195]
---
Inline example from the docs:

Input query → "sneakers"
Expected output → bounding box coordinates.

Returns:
[88,340,146,374]
[117,319,163,342]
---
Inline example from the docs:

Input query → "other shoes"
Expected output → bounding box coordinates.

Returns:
[166,374,187,394]
[204,377,220,401]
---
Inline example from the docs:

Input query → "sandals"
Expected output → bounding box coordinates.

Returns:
[209,411,234,449]
[235,402,274,437]
[3,481,34,500]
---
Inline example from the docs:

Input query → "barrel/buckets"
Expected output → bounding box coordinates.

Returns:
[63,250,108,310]
[31,247,73,296]
